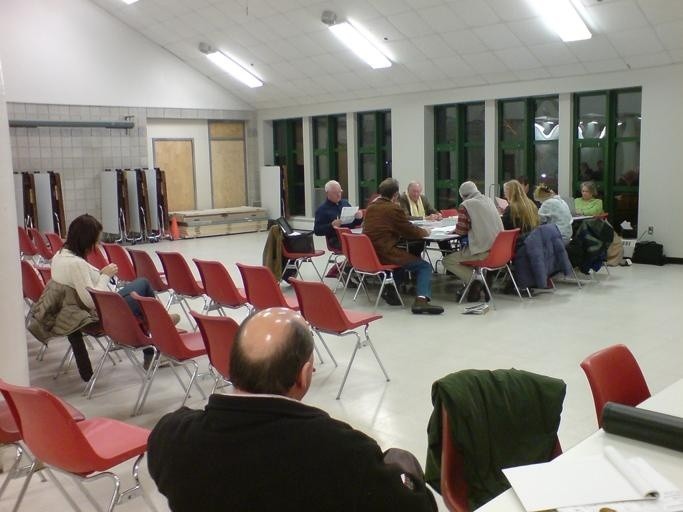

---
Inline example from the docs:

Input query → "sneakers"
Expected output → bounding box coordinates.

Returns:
[412,301,444,313]
[337,273,357,288]
[382,285,401,305]
[468,281,491,302]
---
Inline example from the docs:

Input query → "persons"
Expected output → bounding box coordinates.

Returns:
[399,182,456,276]
[362,179,444,313]
[533,184,573,247]
[51,214,181,370]
[517,175,542,209]
[315,180,365,287]
[574,180,603,217]
[501,179,540,293]
[442,180,505,302]
[147,307,440,511]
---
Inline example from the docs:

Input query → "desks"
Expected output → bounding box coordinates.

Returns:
[475,377,683,511]
[259,165,286,229]
[13,167,173,245]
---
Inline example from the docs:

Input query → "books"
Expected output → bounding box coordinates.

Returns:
[500,444,659,512]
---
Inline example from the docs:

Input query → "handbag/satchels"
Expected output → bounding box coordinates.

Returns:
[632,241,663,265]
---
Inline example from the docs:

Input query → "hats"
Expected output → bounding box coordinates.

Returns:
[458,180,478,200]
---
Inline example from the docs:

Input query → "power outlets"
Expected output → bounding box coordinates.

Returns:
[648,225,653,234]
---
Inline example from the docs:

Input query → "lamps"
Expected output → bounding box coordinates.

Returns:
[321,10,392,70]
[199,42,263,88]
[525,0,592,42]
